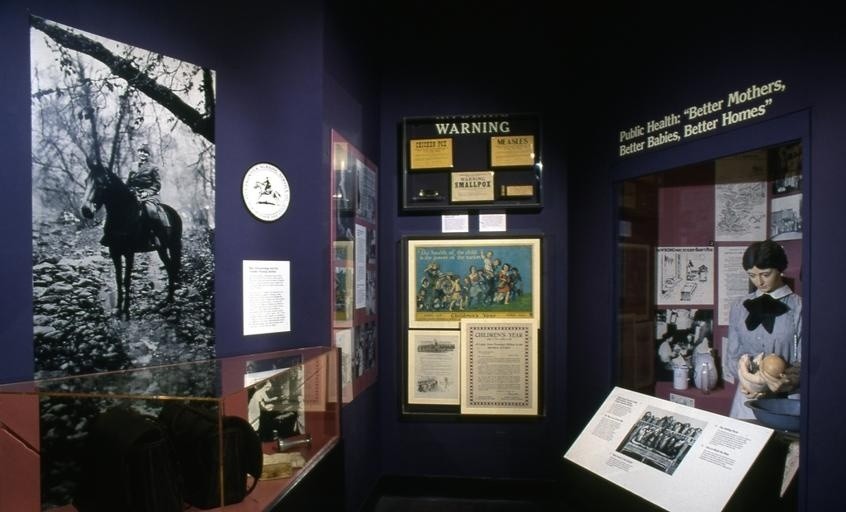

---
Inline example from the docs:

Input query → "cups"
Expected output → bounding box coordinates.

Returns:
[673,365,691,390]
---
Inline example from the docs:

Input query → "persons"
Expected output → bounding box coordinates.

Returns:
[246,377,278,435]
[656,308,715,369]
[416,247,520,312]
[332,148,379,385]
[726,241,802,429]
[121,141,172,252]
[625,410,703,462]
[736,353,786,399]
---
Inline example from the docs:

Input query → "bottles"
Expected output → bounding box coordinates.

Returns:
[700,362,712,395]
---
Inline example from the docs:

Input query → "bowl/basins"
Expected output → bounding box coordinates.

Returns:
[743,398,800,433]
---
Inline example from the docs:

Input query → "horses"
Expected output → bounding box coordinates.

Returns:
[79,157,183,321]
[254,181,281,200]
